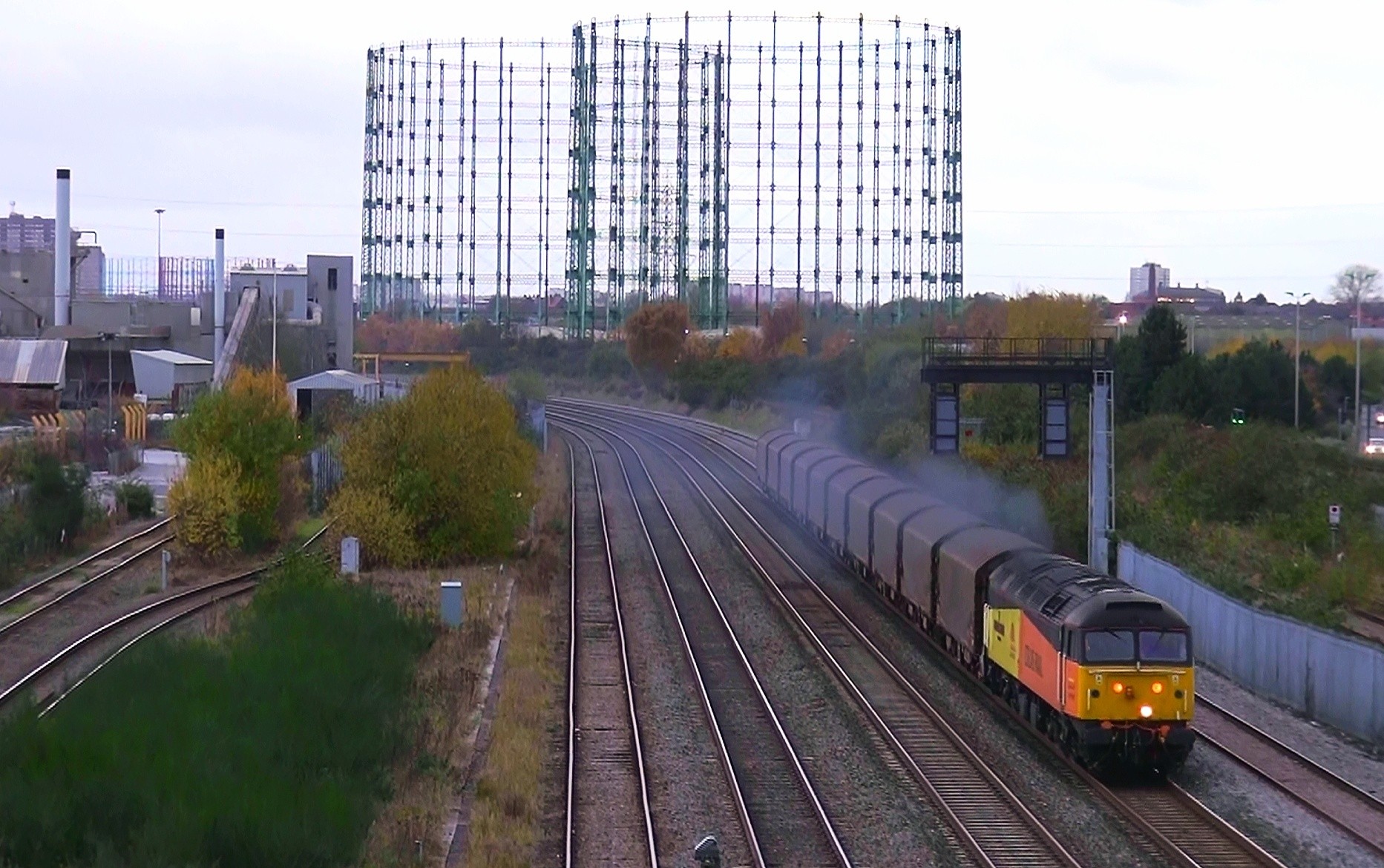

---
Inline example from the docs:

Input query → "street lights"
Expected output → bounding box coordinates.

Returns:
[1285,290,1311,432]
[98,330,118,437]
[1344,272,1378,452]
[155,209,167,301]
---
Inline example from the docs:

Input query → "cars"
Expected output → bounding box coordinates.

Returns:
[1364,437,1384,454]
[1374,406,1384,424]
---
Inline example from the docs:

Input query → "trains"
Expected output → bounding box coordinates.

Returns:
[754,428,1198,778]
